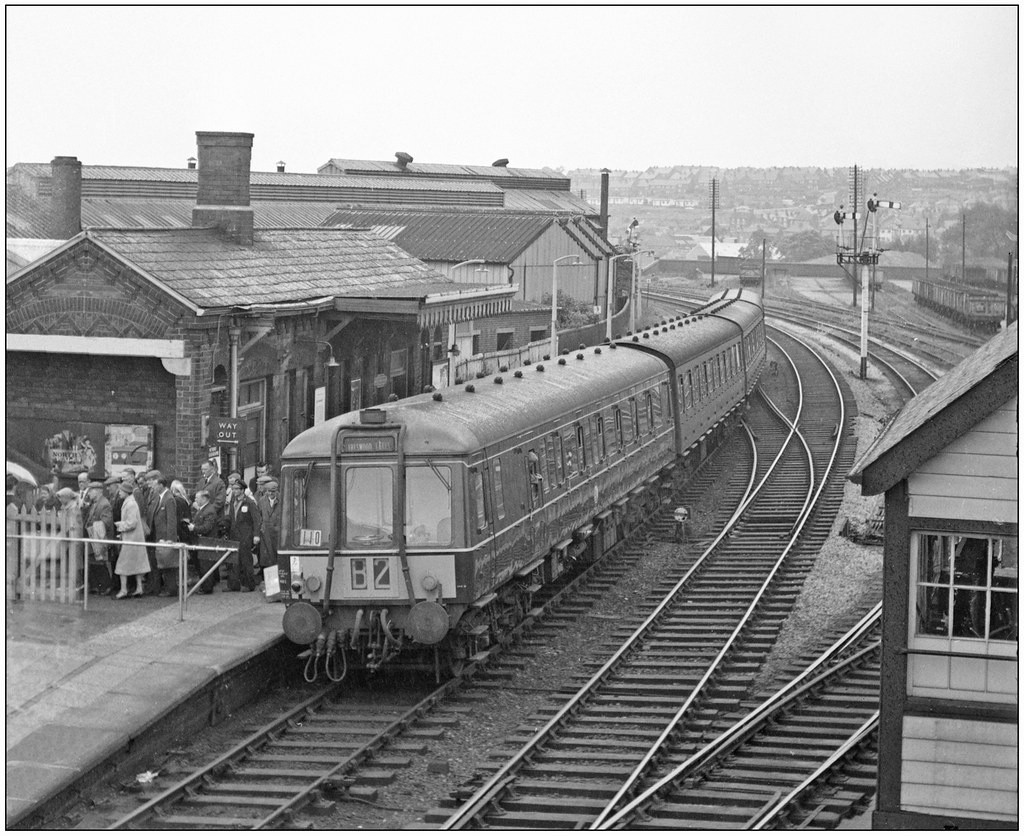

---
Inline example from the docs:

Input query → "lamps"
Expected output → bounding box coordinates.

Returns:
[294,339,340,367]
[447,323,459,352]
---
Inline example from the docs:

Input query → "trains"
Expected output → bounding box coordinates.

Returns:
[276,286,767,686]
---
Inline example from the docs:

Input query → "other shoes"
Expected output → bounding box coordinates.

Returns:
[251,546,264,585]
[100,565,254,600]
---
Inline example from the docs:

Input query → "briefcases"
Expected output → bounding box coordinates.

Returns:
[155,547,190,568]
[198,536,241,565]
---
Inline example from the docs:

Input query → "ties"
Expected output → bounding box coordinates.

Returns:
[80,492,85,500]
[205,479,207,485]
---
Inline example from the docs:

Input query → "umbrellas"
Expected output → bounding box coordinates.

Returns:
[7,461,37,489]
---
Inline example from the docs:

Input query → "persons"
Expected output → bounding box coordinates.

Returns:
[2,461,281,595]
[114,482,152,599]
[223,478,261,592]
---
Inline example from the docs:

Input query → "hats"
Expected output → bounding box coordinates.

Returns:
[88,481,103,488]
[265,482,278,491]
[230,479,247,489]
[104,477,120,485]
[145,470,160,480]
[116,482,134,493]
[256,475,272,484]
